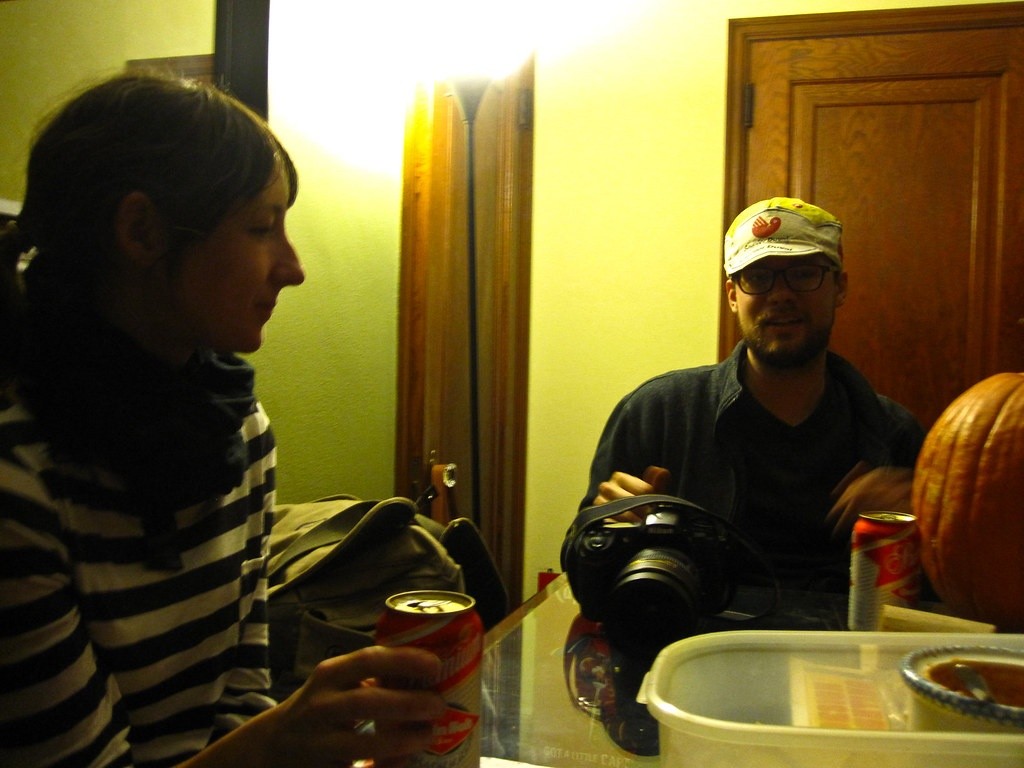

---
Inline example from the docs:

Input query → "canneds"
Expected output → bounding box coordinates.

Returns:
[371,589,483,767]
[845,511,918,632]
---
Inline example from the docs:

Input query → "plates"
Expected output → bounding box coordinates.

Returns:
[897,639,1024,728]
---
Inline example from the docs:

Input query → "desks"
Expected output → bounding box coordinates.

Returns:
[354,573,847,767]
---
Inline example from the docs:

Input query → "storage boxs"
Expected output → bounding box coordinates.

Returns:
[639,632,1023,767]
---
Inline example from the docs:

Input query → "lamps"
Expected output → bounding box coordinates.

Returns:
[418,0,535,519]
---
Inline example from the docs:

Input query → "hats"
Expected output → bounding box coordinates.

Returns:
[723,196,844,275]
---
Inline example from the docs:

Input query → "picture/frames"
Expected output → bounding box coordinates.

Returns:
[127,52,214,88]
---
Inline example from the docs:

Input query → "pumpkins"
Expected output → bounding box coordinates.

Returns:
[911,371,1024,629]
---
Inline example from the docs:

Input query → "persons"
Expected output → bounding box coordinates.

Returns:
[578,196,939,611]
[0,76,446,768]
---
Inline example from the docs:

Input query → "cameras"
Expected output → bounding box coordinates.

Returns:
[567,505,739,639]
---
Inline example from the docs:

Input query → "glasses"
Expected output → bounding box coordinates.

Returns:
[734,265,840,293]
